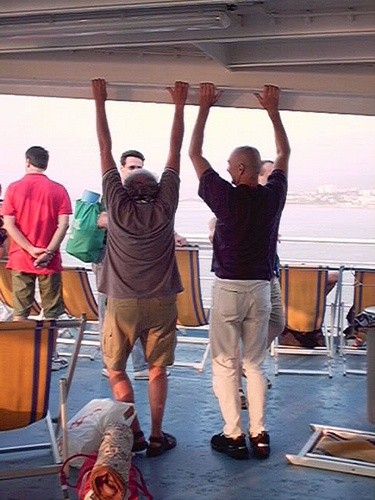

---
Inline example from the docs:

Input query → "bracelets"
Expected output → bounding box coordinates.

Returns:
[46,250,55,257]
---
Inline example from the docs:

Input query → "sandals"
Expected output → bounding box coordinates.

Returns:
[130,432,148,457]
[148,432,177,457]
[238,388,248,408]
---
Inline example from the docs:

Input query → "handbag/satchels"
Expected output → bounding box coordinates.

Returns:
[65,197,105,264]
[60,449,154,500]
[55,398,137,469]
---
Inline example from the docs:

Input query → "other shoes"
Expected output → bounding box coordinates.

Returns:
[211,430,249,460]
[51,356,69,371]
[248,432,270,460]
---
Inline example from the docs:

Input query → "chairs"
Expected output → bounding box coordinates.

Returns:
[268,263,338,379]
[143,243,210,372]
[0,312,87,500]
[335,265,375,377]
[0,257,42,320]
[55,266,100,361]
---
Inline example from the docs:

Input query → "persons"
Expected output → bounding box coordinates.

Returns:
[239,160,286,409]
[92,78,189,456]
[97,152,171,379]
[188,82,291,461]
[0,146,73,370]
